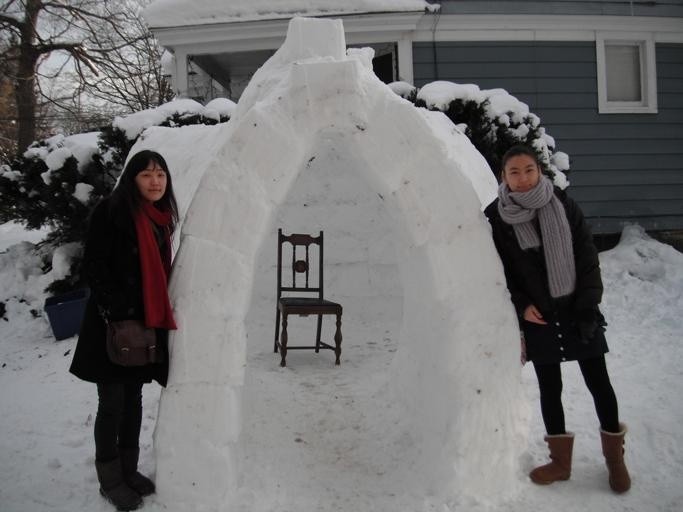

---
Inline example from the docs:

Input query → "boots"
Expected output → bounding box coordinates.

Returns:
[530,434,573,484]
[95,448,155,510]
[599,423,631,492]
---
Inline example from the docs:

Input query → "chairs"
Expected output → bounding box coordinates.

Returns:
[269,227,346,366]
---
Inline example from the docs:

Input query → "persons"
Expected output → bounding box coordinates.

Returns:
[67,148,179,511]
[482,144,631,494]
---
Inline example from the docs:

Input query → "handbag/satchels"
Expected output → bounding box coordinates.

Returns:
[106,320,169,366]
